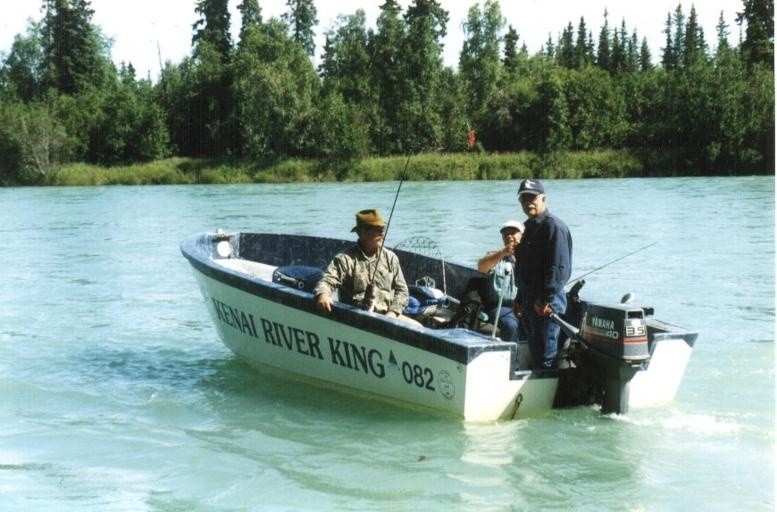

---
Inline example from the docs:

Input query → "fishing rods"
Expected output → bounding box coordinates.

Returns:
[359,135,415,310]
[472,235,660,332]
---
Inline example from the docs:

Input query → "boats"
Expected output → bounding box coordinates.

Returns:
[179,225,700,425]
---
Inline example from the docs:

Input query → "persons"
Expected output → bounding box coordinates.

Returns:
[511,179,571,371]
[477,221,524,370]
[315,208,424,328]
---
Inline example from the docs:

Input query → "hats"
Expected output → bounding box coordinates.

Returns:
[500,220,525,234]
[350,210,388,232]
[517,178,545,196]
